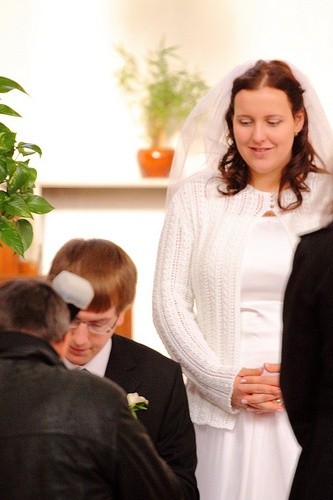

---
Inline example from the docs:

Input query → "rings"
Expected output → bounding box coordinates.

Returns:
[277,399,281,405]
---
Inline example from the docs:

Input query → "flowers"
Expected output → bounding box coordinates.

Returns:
[127,392,149,419]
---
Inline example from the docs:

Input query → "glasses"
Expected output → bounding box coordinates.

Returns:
[70,315,119,335]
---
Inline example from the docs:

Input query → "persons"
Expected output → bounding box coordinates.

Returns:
[278,220,333,500]
[152,59,333,500]
[44,237,202,500]
[0,277,185,500]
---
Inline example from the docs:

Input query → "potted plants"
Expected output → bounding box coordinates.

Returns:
[113,32,211,179]
[0,76,55,287]
